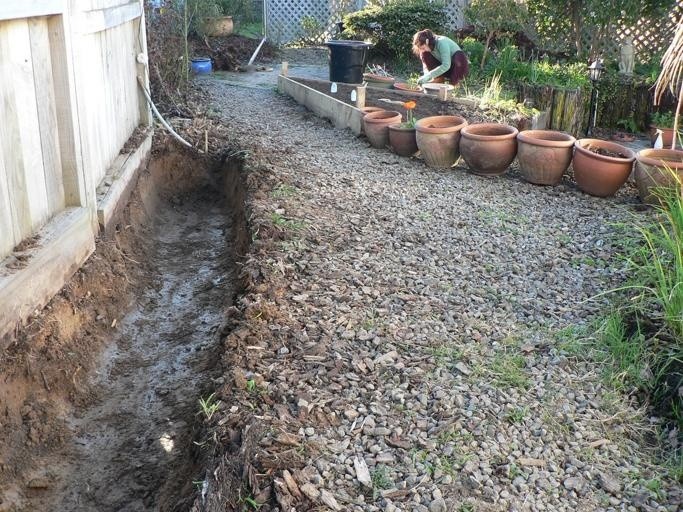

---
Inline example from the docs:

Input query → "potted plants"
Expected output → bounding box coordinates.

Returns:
[204,3,233,38]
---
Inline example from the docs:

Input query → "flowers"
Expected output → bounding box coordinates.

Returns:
[398,99,417,129]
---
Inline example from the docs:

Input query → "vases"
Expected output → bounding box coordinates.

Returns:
[413,116,682,209]
[192,58,214,75]
[361,107,416,156]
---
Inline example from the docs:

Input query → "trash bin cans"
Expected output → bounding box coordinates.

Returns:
[324,40,373,84]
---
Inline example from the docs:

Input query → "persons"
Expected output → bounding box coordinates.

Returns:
[412,29,471,88]
